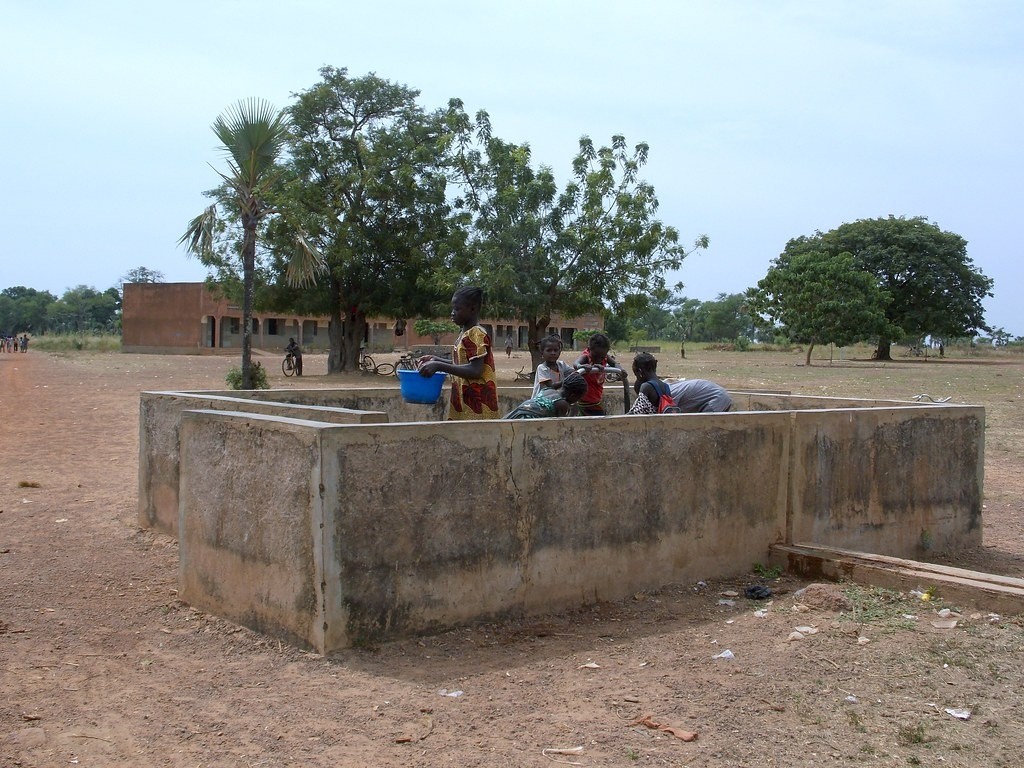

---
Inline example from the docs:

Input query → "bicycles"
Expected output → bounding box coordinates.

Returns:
[870,351,878,359]
[360,347,430,380]
[282,348,298,377]
[906,347,924,358]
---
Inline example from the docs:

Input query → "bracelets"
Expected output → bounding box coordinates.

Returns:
[577,363,581,369]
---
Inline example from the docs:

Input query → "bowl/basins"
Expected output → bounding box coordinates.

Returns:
[396,369,449,405]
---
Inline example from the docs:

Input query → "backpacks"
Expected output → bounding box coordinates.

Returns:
[647,381,681,413]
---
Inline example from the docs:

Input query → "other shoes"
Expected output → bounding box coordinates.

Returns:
[286,367,292,370]
[297,373,302,376]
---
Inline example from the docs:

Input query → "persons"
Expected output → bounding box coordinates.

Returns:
[634,378,733,413]
[625,353,671,415]
[417,285,500,421]
[573,332,628,416]
[0,334,30,353]
[284,337,303,376]
[504,334,513,358]
[501,333,588,419]
